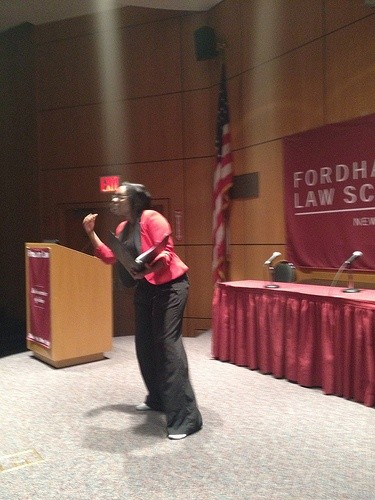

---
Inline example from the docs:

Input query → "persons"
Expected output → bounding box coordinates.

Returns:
[81,182,203,439]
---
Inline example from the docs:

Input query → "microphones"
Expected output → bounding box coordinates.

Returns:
[264,252,281,265]
[344,250,362,266]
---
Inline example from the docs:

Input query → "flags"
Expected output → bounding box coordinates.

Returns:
[212,50,236,288]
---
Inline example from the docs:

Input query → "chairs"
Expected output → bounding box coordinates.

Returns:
[272,261,297,283]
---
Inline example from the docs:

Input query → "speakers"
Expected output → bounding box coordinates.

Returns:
[194,27,217,61]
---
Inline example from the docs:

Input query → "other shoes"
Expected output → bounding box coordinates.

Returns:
[136,403,152,410]
[168,434,187,440]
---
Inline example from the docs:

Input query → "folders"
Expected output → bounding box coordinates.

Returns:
[105,232,169,280]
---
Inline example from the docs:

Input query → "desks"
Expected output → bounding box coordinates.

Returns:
[212,280,375,409]
